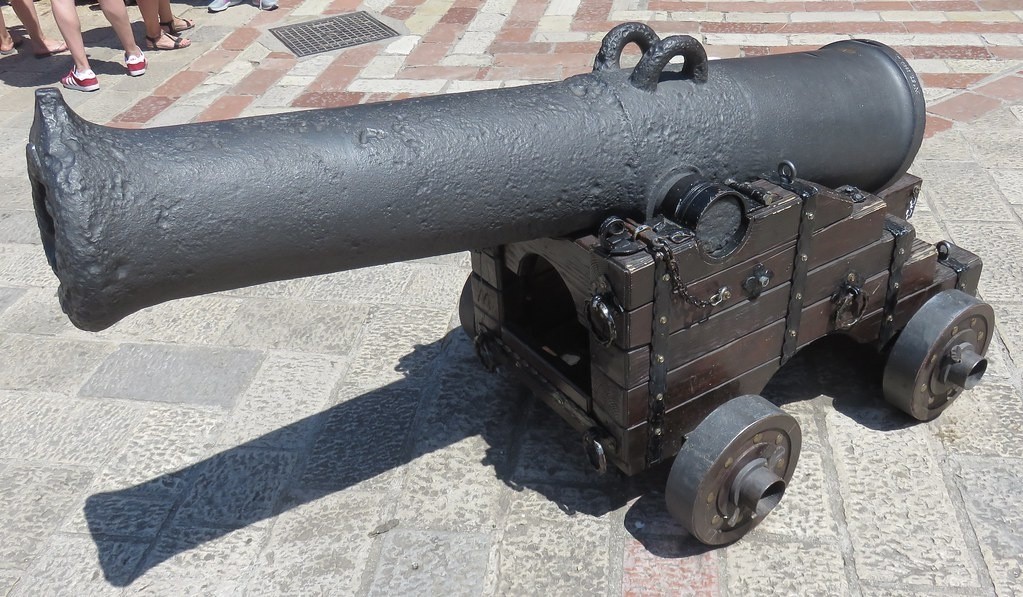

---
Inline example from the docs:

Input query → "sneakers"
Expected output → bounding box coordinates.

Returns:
[208,0,243,12]
[252,0,279,10]
[60,64,100,92]
[124,46,148,77]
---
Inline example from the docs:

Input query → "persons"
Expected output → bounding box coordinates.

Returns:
[50,0,148,91]
[136,0,195,49]
[0,0,69,55]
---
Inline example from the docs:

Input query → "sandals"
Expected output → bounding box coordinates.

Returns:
[159,15,196,35]
[145,31,191,51]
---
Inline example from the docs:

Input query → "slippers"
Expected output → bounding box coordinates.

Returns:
[34,40,69,59]
[0,35,24,55]
